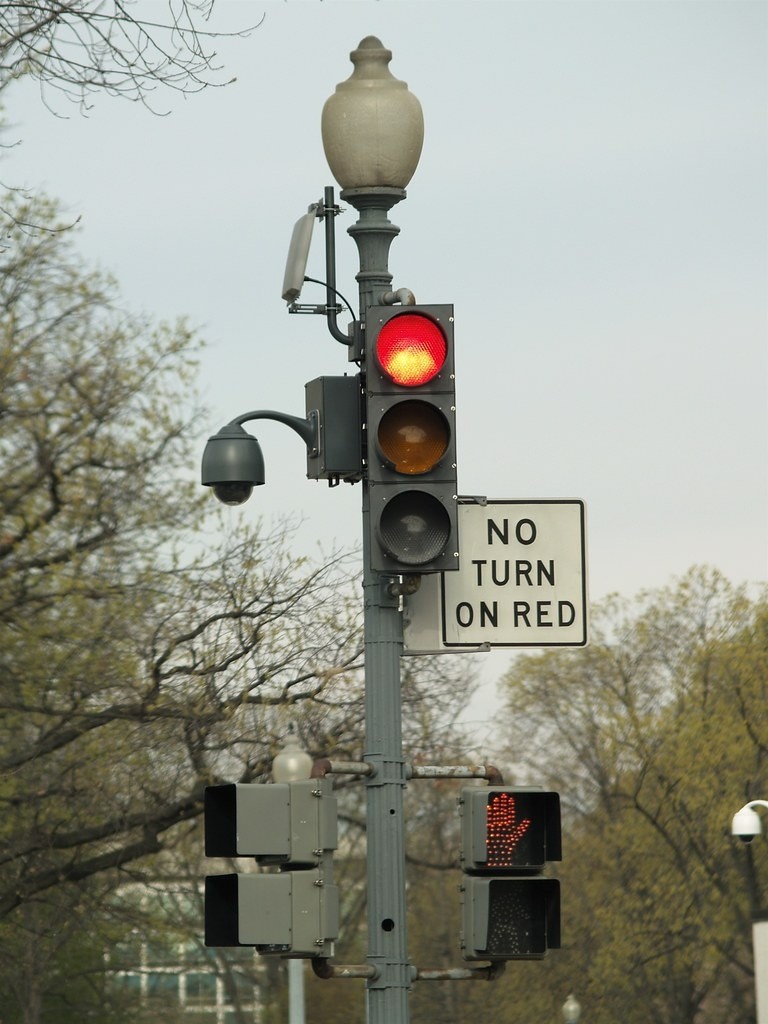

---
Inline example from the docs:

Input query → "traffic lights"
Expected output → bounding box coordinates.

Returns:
[362,303,462,576]
[204,777,342,958]
[457,784,564,964]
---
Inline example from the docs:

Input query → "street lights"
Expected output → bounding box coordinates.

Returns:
[316,29,424,1022]
[562,995,581,1024]
[266,742,314,1024]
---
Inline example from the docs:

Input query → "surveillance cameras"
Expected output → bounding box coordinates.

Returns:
[731,809,762,843]
[200,434,266,506]
